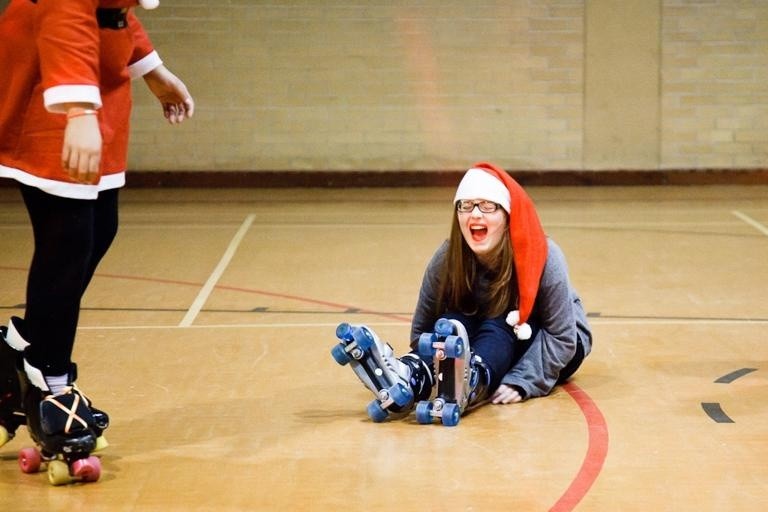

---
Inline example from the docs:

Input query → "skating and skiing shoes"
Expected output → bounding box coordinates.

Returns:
[0,316,109,484]
[415,318,487,426]
[331,323,432,422]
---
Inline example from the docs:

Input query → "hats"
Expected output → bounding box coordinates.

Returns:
[454,161,547,340]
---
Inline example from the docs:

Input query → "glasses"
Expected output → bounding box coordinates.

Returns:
[456,200,501,213]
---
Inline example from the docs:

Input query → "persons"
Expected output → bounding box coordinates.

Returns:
[1,2,195,455]
[350,163,592,411]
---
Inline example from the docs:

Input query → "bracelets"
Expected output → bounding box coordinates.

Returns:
[65,109,101,122]
